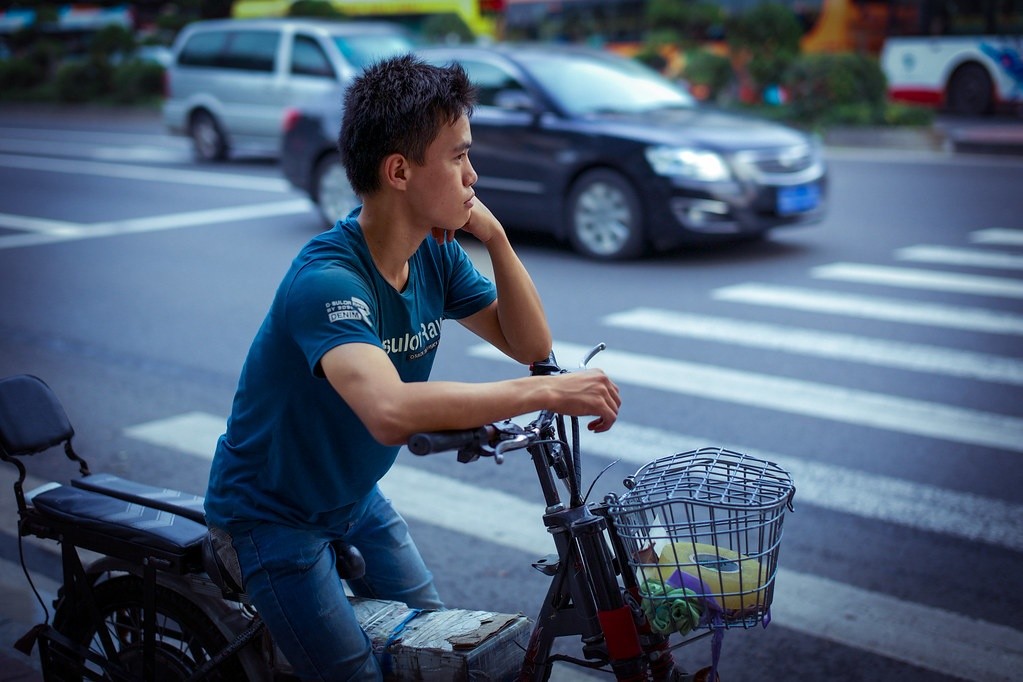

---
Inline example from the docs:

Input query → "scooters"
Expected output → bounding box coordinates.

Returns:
[0,343,800,682]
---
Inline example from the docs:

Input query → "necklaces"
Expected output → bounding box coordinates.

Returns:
[205,54,622,681]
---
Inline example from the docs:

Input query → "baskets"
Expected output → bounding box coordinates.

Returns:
[607,447,796,628]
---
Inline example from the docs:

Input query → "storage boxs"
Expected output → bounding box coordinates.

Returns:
[273,596,531,682]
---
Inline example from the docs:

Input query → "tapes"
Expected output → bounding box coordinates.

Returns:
[652,543,768,610]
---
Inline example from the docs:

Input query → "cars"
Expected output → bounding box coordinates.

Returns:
[277,42,832,264]
[157,18,437,165]
